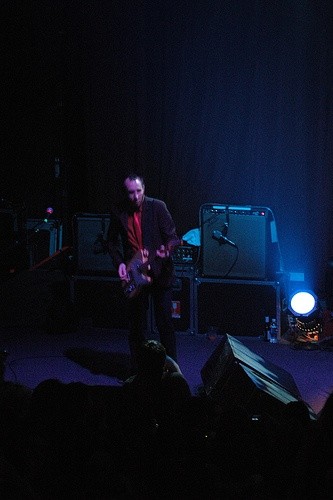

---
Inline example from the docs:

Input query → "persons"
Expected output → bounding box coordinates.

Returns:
[317,298,333,319]
[106,174,180,383]
[0,379,333,500]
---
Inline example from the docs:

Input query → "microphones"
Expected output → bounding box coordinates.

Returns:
[211,230,237,248]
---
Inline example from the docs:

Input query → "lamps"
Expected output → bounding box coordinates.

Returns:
[287,288,329,350]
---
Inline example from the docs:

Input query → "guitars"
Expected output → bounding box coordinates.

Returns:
[122,240,186,298]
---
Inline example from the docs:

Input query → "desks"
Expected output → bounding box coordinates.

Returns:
[70,274,152,332]
[193,277,286,341]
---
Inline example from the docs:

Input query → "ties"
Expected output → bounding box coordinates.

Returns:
[133,210,143,250]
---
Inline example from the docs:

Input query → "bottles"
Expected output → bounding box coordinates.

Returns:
[263,316,279,344]
[172,246,192,263]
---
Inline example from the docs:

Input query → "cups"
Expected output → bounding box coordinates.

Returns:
[207,327,218,342]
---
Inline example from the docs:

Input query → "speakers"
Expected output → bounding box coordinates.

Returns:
[76,217,126,276]
[208,359,307,430]
[200,334,302,399]
[200,204,271,280]
